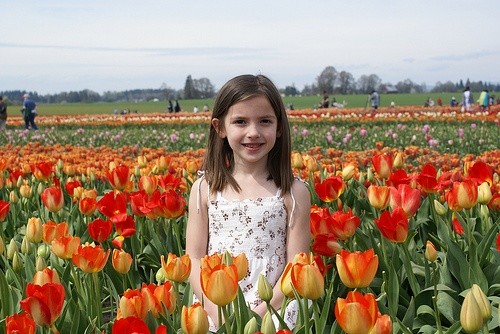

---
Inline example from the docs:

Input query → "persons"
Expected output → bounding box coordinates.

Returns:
[320,91,329,108]
[368,90,378,109]
[167,100,173,113]
[202,103,210,113]
[193,105,199,113]
[0,96,7,131]
[21,92,38,130]
[488,95,500,106]
[174,100,180,113]
[425,97,442,107]
[462,86,475,112]
[450,97,458,107]
[331,97,337,107]
[476,89,489,111]
[186,75,310,333]
